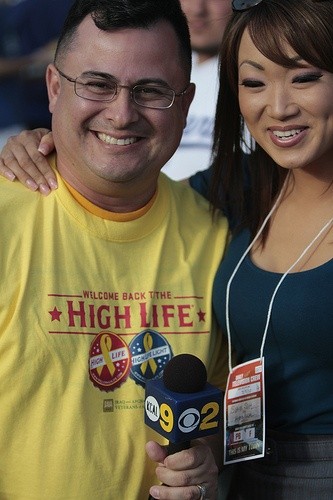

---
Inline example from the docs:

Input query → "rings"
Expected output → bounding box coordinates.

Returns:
[196,483,208,500]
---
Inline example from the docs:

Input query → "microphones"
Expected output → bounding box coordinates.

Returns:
[143,353,224,457]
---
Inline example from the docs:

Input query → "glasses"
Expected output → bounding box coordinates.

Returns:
[231,0,261,11]
[56,67,188,110]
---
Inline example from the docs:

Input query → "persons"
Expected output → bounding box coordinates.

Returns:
[0,0,237,500]
[0,0,333,500]
[160,0,256,181]
